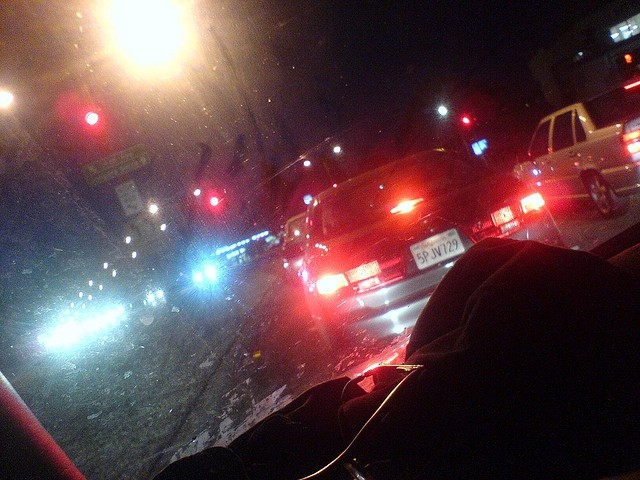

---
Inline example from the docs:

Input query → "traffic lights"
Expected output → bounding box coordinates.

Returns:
[205,191,223,213]
[79,107,106,136]
[457,111,474,131]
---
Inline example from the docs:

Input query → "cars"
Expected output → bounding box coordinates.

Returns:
[513,77,640,219]
[299,148,562,355]
[282,211,306,286]
